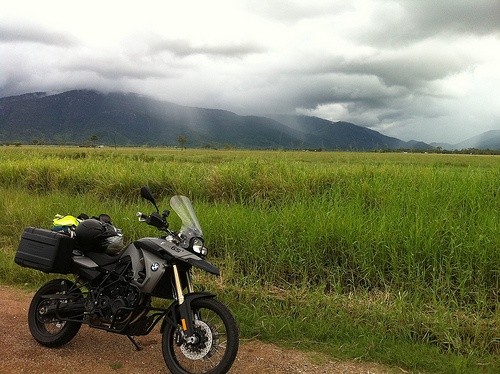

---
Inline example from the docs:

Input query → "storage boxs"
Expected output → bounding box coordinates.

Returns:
[14,225,75,274]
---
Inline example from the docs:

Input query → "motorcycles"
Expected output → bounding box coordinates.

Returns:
[13,186,238,374]
